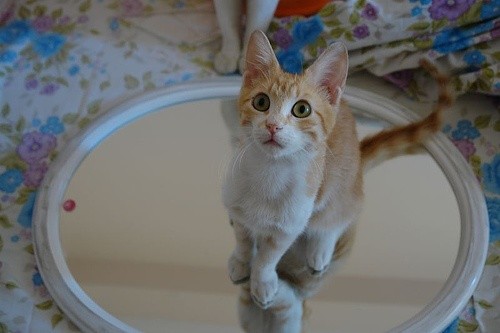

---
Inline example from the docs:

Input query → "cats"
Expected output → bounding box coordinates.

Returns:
[212,0,278,75]
[230,228,354,333]
[222,30,455,309]
